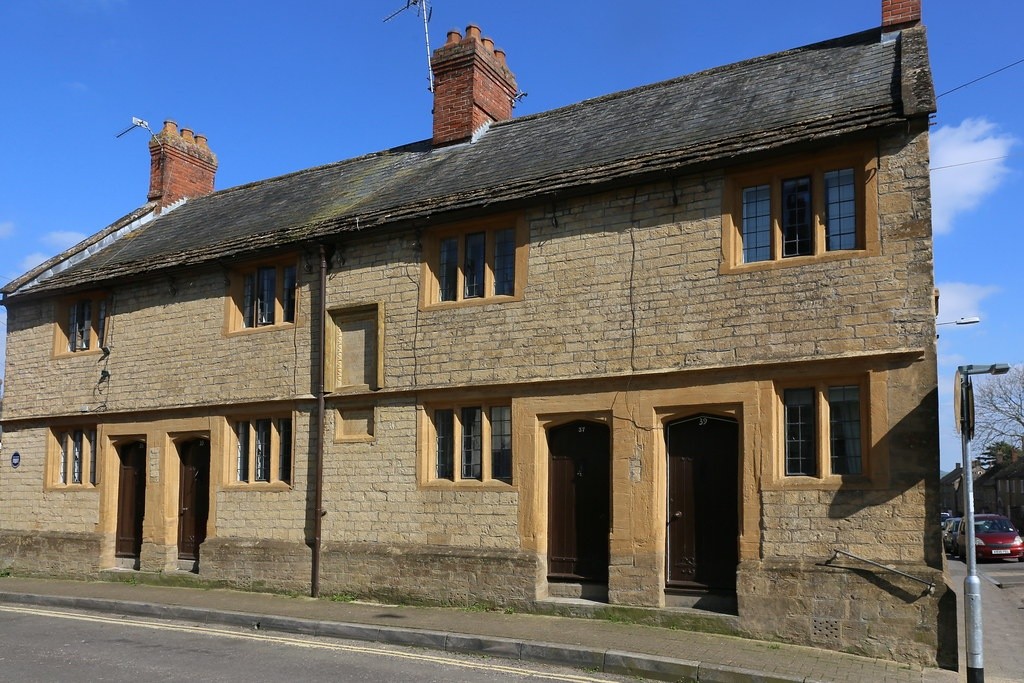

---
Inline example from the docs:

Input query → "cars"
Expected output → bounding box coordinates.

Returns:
[939,512,1024,564]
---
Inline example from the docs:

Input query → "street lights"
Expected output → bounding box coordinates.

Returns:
[956,362,1012,682]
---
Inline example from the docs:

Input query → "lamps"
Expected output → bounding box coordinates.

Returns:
[101,370,112,377]
[101,346,110,354]
